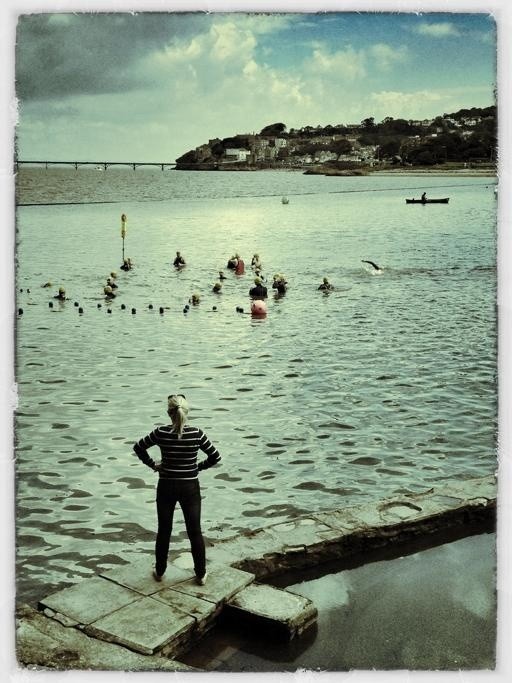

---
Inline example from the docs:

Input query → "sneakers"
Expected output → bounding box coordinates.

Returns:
[152,567,166,582]
[195,569,208,586]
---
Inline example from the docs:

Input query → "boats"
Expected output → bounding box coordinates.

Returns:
[406,198,450,203]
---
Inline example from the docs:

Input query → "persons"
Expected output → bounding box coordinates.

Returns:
[254,268,266,280]
[54,286,69,300]
[189,292,202,304]
[213,282,223,293]
[121,260,130,270]
[104,285,115,300]
[317,276,335,289]
[106,276,118,289]
[421,191,428,199]
[227,255,237,266]
[173,251,185,265]
[229,253,245,275]
[219,271,228,278]
[271,272,287,296]
[132,393,223,585]
[248,276,268,300]
[252,252,261,267]
[126,256,135,268]
[111,270,119,278]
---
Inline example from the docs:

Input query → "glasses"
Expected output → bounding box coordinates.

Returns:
[168,394,186,399]
